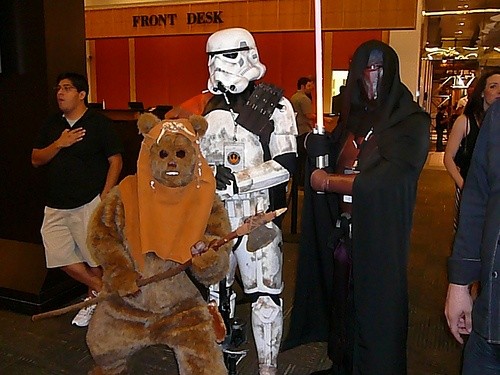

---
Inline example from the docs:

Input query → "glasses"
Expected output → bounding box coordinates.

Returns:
[56,85,75,92]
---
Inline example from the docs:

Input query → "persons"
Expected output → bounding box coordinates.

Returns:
[444,96,500,375]
[31,73,124,326]
[442,71,500,236]
[435,107,445,152]
[182,28,299,375]
[291,77,316,190]
[294,38,433,375]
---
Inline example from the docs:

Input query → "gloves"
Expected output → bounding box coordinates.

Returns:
[307,133,331,157]
[215,166,235,189]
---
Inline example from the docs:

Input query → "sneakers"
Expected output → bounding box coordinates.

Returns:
[71,297,98,327]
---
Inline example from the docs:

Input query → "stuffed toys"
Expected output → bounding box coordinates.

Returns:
[85,111,233,375]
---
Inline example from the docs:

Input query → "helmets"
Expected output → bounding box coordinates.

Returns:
[207,28,266,95]
